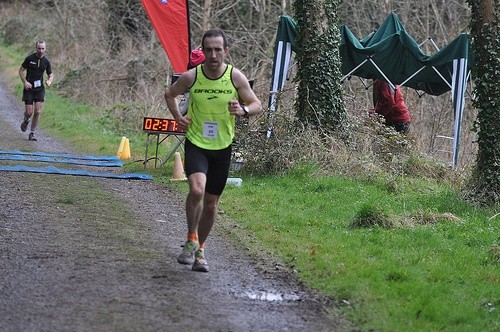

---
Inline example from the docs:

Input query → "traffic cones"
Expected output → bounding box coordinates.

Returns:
[170,152,188,181]
[117,137,130,159]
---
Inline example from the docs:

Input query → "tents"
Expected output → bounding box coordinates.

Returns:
[266,11,473,170]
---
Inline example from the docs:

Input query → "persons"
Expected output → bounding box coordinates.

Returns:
[18,39,55,141]
[373,78,412,136]
[163,29,263,273]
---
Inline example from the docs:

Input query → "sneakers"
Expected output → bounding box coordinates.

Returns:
[190,248,211,272]
[175,238,199,265]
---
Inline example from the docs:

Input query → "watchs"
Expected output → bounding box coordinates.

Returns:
[242,106,249,117]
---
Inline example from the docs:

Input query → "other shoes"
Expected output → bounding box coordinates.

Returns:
[20,120,29,132]
[28,132,37,142]
